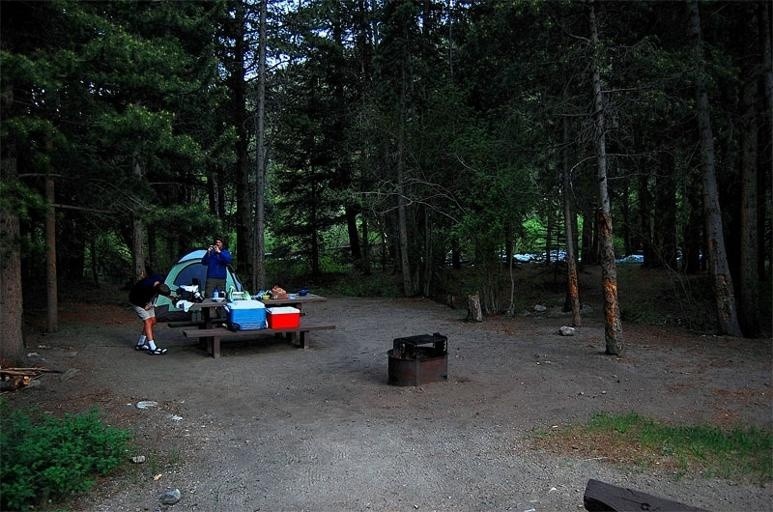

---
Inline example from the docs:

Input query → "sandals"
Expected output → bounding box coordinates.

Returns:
[135,342,168,355]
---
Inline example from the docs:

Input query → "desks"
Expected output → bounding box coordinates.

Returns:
[174,292,327,354]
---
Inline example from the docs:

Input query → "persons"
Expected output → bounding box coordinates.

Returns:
[200,236,233,297]
[125,273,176,356]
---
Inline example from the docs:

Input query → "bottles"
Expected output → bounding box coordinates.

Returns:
[213,290,219,298]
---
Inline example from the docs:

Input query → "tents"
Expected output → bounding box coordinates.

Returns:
[150,250,245,323]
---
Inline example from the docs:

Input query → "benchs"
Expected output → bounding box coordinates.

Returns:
[168,311,305,345]
[183,322,336,359]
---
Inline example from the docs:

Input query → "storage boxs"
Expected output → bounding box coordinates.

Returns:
[223,299,266,331]
[266,306,301,329]
